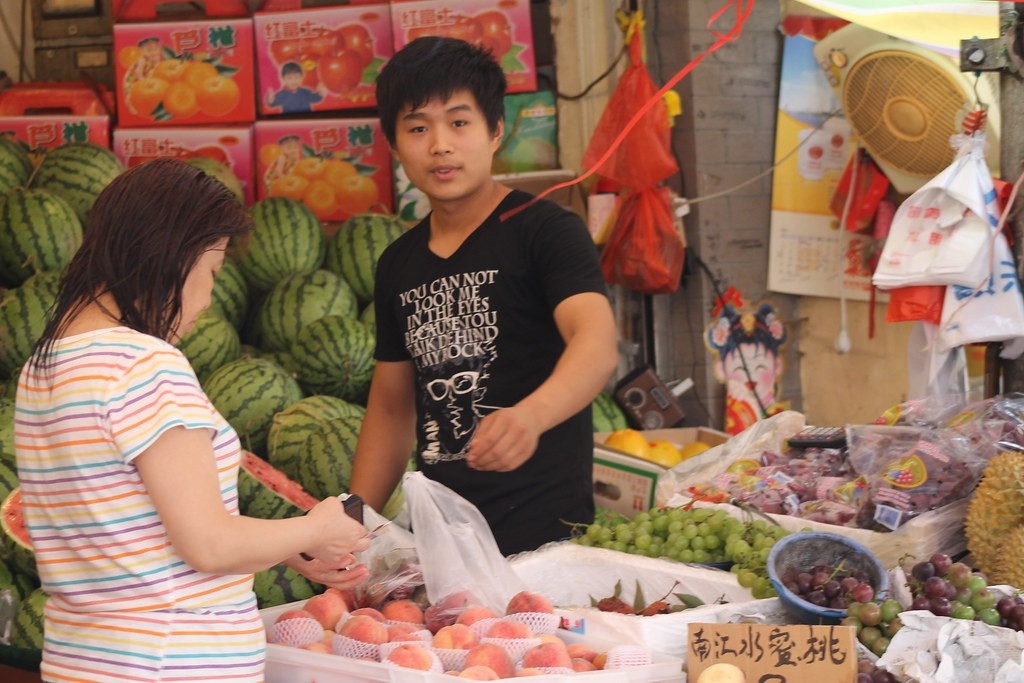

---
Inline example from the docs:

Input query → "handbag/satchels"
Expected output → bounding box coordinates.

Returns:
[583,21,679,190]
[600,185,684,293]
[402,471,525,616]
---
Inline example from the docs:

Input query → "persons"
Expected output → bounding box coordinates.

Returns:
[265,63,326,113]
[13,157,368,683]
[351,36,620,559]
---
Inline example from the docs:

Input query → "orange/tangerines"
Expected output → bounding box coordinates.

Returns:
[603,428,712,470]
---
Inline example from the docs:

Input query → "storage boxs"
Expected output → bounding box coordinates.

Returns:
[253,4,393,114]
[113,130,256,208]
[112,19,256,128]
[0,115,110,159]
[394,90,559,225]
[391,0,536,98]
[254,119,395,226]
[260,413,1024,683]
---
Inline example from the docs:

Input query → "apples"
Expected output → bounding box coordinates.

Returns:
[127,11,515,223]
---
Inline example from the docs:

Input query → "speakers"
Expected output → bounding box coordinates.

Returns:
[613,364,684,430]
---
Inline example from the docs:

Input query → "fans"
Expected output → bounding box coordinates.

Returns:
[814,23,1000,191]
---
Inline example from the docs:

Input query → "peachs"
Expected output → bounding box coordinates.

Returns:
[268,585,654,681]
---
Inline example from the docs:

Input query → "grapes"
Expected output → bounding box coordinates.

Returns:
[780,552,1024,683]
[565,496,821,601]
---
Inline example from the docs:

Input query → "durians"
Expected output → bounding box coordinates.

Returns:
[962,452,1024,594]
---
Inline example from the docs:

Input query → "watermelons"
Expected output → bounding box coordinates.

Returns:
[0,133,423,650]
[592,389,629,431]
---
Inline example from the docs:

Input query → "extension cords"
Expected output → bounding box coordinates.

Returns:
[669,192,689,249]
